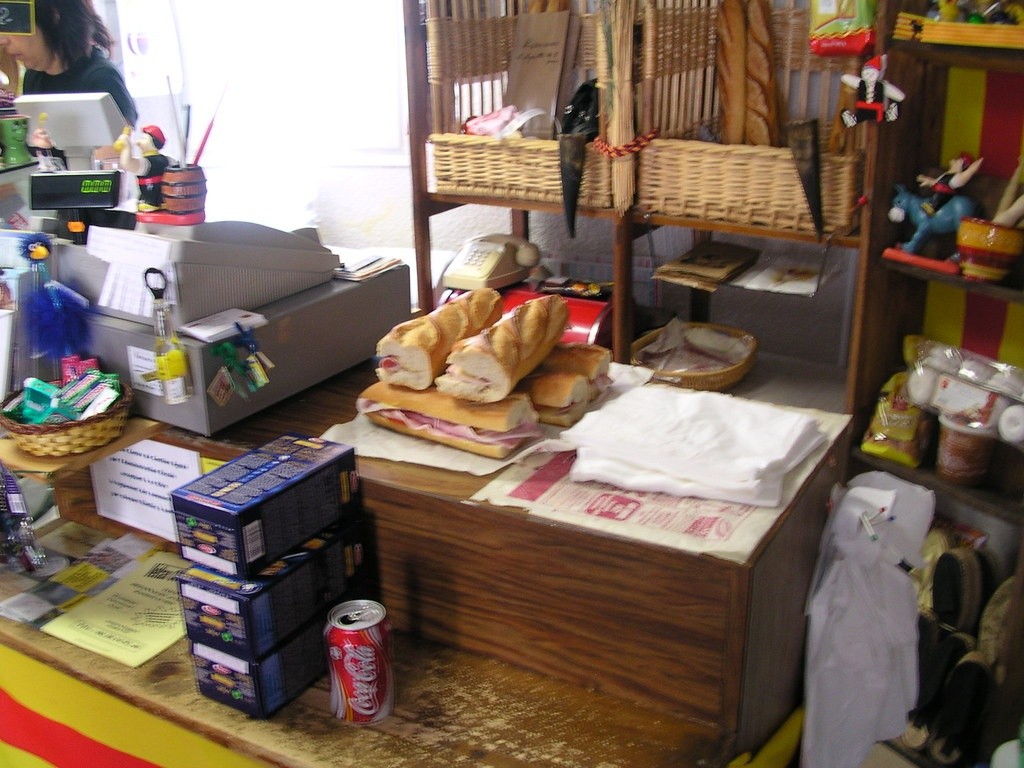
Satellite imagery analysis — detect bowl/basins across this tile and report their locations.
[958,216,1024,282]
[935,417,996,486]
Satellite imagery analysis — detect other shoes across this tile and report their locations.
[901,631,976,750]
[929,649,993,765]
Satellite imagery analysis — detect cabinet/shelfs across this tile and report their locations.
[850,0,1024,581]
[403,0,951,442]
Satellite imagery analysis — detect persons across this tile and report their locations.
[118,125,165,211]
[0,0,138,129]
[917,152,984,214]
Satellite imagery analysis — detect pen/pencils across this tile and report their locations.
[164,74,229,170]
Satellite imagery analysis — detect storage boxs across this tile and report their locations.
[175,504,380,658]
[170,430,362,580]
[189,569,385,722]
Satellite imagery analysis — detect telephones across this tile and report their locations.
[440,234,542,290]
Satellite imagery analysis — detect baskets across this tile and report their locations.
[0,372,136,459]
[636,0,864,236]
[630,321,758,392]
[426,0,617,208]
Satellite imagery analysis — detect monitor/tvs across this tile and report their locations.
[13,92,134,171]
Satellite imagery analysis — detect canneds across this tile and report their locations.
[323,600,396,726]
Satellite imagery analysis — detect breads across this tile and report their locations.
[525,0,569,14]
[714,0,781,149]
[354,287,612,459]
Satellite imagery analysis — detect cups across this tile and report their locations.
[0,116,32,163]
[162,164,207,214]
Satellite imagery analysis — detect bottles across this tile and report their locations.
[145,268,192,406]
